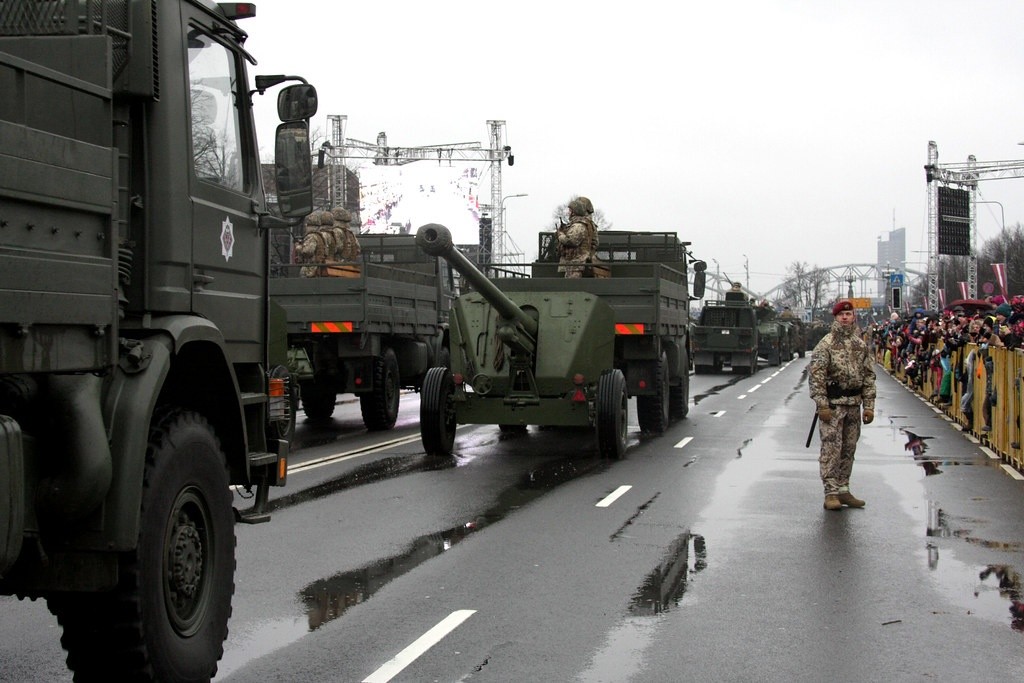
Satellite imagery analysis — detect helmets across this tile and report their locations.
[333,206,352,222]
[568,201,587,216]
[321,211,334,225]
[305,213,321,227]
[575,197,594,213]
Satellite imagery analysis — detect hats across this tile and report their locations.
[995,303,1012,317]
[832,301,854,314]
[954,305,964,311]
[989,296,1004,306]
[983,317,993,327]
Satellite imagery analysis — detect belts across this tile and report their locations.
[829,387,863,397]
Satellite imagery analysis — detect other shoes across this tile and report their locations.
[841,491,866,508]
[982,425,993,432]
[962,425,974,432]
[929,393,952,406]
[823,494,842,511]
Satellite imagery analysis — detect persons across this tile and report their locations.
[870,295,1024,449]
[294,207,361,278]
[781,306,795,318]
[556,196,599,278]
[809,301,877,509]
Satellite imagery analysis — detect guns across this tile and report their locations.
[290,232,304,242]
[559,216,566,228]
[805,404,819,448]
[722,271,734,287]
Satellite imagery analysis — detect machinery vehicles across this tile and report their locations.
[687,294,805,376]
[525,228,708,437]
[267,225,462,431]
[0,0,319,683]
[411,221,636,463]
